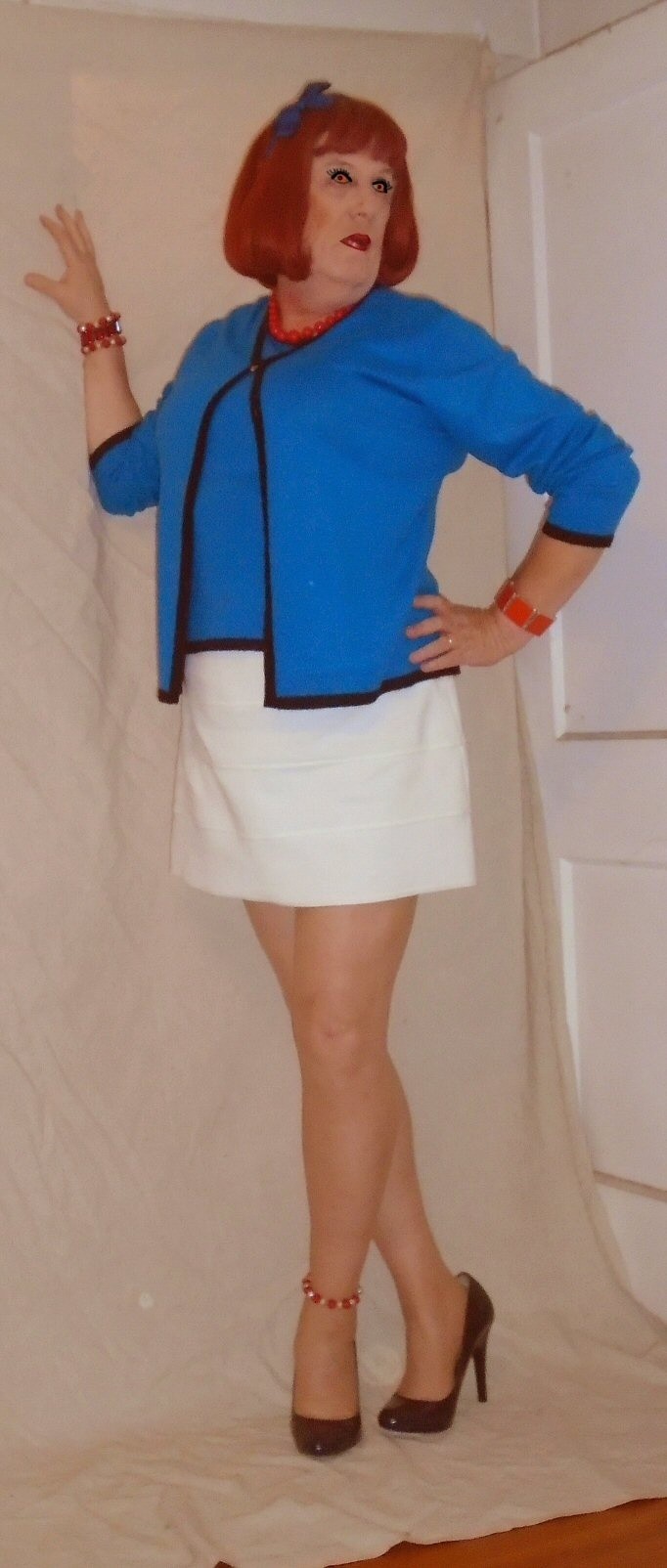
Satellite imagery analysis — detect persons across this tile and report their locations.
[24,83,640,1457]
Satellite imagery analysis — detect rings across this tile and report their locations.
[441,633,454,648]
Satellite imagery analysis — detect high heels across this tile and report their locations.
[380,1270,491,1431]
[290,1340,363,1457]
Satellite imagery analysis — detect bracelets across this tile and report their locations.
[79,313,127,354]
[494,579,556,638]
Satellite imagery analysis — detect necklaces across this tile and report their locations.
[268,286,354,343]
